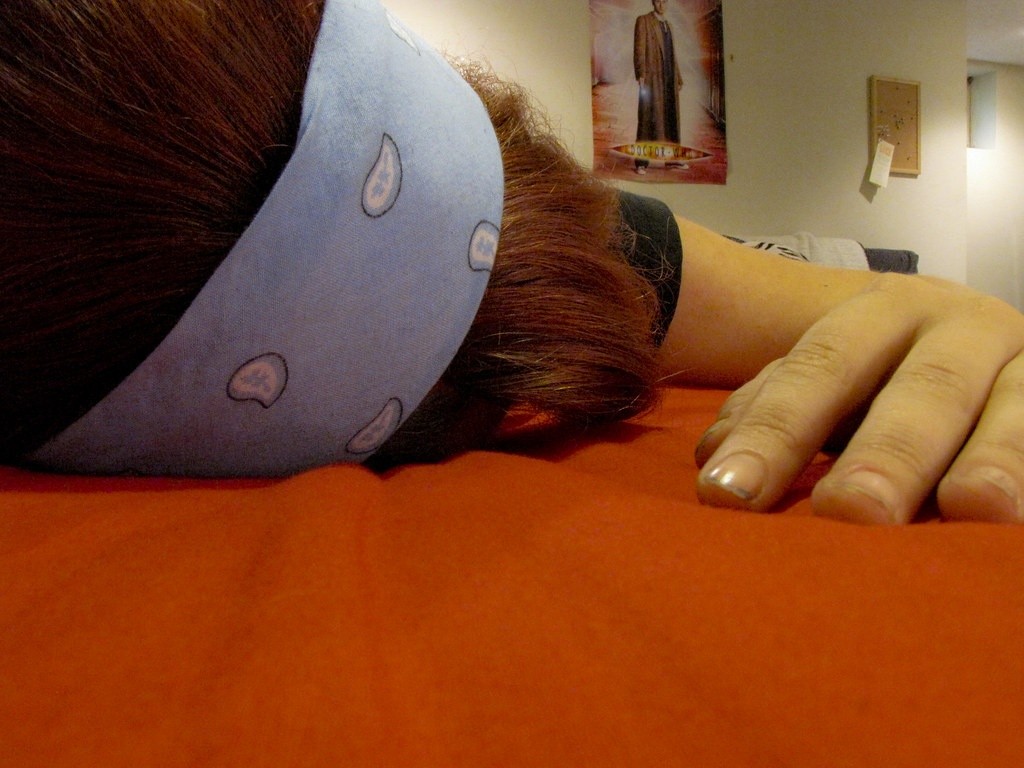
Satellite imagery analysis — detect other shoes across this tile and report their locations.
[637,166,646,175]
[665,163,689,169]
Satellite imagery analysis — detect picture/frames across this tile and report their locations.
[870,75,921,175]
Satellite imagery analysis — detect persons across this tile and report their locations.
[635,0,690,174]
[1,0,1024,525]
